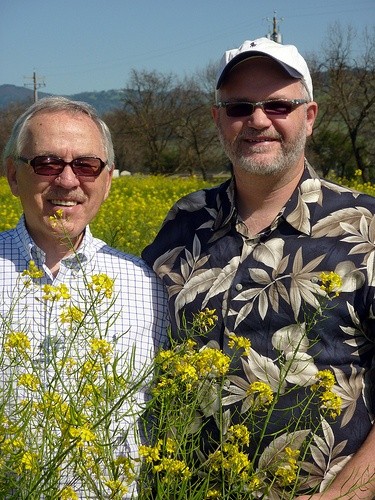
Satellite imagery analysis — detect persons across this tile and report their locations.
[141,37,375,500]
[0,96,171,500]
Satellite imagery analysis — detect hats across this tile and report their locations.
[216,37,313,102]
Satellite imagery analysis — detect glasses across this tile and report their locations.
[217,99,308,117]
[17,155,109,176]
